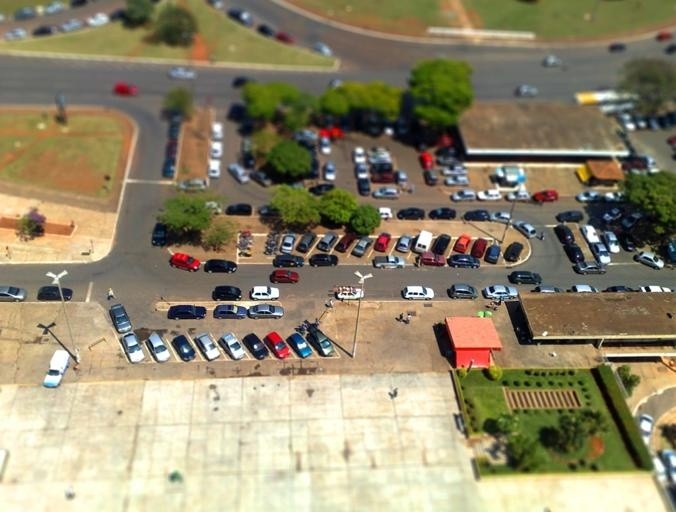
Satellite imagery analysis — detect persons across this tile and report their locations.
[399,313,404,322]
[540,232,544,241]
[329,298,334,309]
[298,317,320,332]
[407,313,411,324]
[107,287,116,301]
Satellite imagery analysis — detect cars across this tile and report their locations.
[266,331,290,359]
[170,335,196,362]
[449,254,481,269]
[1,284,74,303]
[167,304,207,321]
[606,285,633,292]
[308,253,338,267]
[274,254,305,268]
[574,261,607,276]
[250,285,280,301]
[419,253,446,267]
[170,253,201,272]
[638,285,672,292]
[637,252,665,271]
[121,330,145,363]
[535,285,564,292]
[109,303,133,333]
[449,283,478,300]
[213,305,247,319]
[195,332,221,361]
[336,286,365,301]
[151,110,676,265]
[287,333,313,359]
[204,259,237,274]
[403,285,435,300]
[214,285,242,301]
[571,284,599,292]
[374,255,406,269]
[309,329,334,357]
[271,269,299,284]
[145,333,170,363]
[242,333,269,360]
[484,284,517,300]
[517,31,675,97]
[509,271,542,285]
[2,0,333,96]
[638,413,676,492]
[220,332,246,360]
[248,303,284,320]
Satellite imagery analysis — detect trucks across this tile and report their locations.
[43,349,69,388]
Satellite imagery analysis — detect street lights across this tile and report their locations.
[352,270,373,357]
[45,269,80,364]
